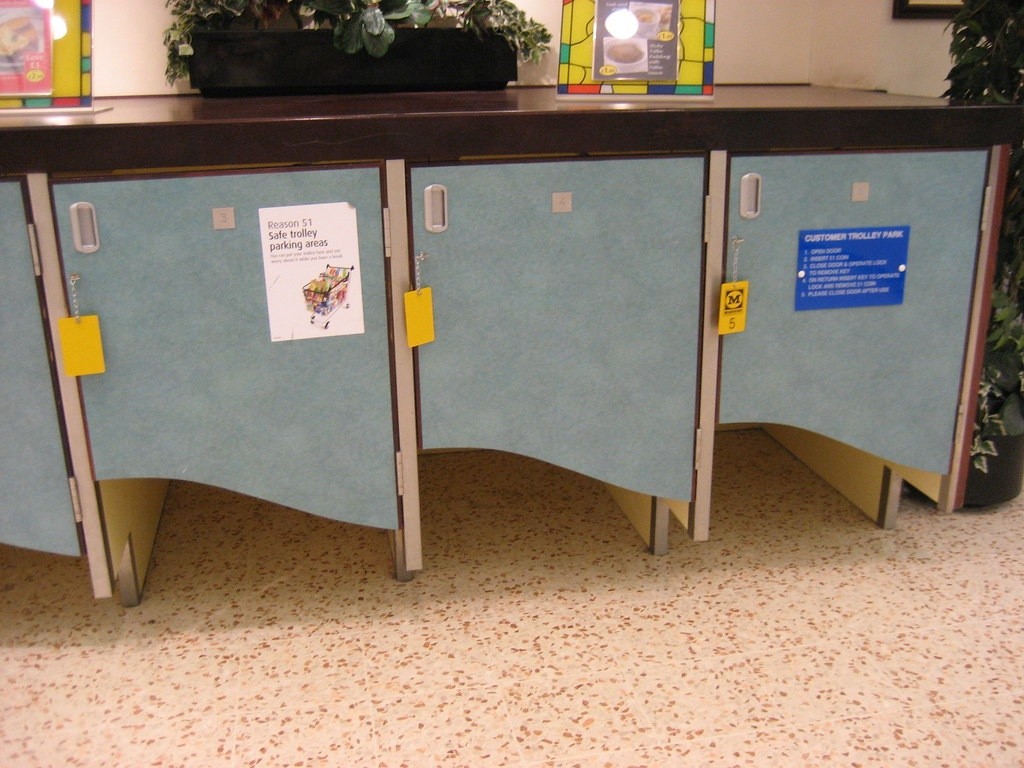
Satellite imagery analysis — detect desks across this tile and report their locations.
[1,82,1023,608]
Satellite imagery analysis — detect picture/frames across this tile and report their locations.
[893,0,966,20]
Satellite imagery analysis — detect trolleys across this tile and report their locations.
[301,263,355,328]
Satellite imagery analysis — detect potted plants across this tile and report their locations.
[939,0,1024,508]
[163,1,554,98]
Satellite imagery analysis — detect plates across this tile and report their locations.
[602,39,647,67]
[0,16,43,67]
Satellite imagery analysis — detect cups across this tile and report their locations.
[633,7,660,34]
